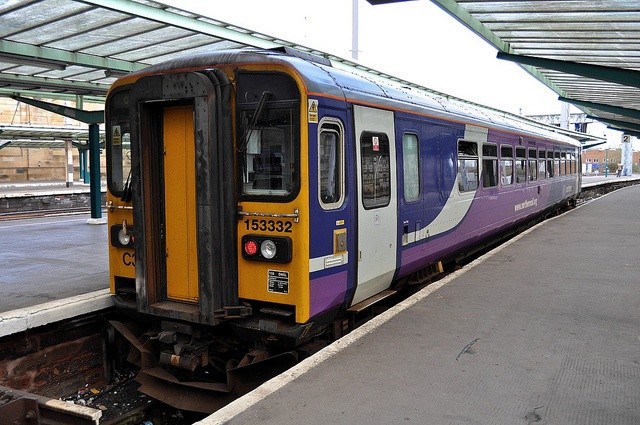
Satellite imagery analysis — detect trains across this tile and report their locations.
[106,48,584,415]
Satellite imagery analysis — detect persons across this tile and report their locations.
[616,162,623,177]
